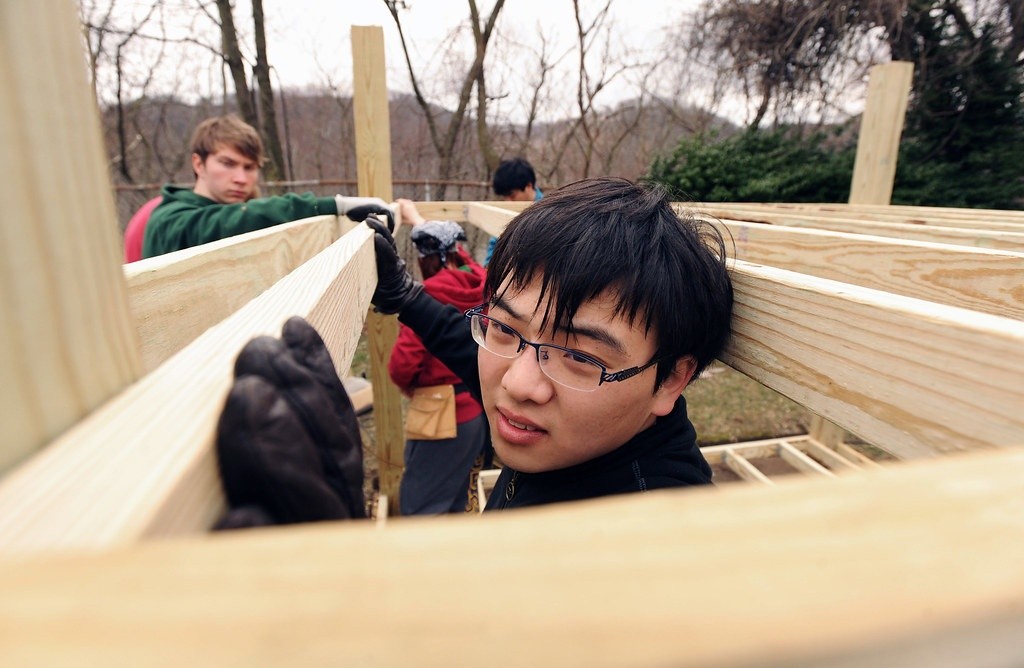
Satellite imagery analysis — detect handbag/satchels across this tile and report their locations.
[405,383,468,440]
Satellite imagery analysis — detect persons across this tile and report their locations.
[139,113,397,262]
[483,157,546,268]
[213,175,734,534]
[384,196,496,515]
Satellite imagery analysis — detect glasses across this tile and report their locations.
[465,301,663,392]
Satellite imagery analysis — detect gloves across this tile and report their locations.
[209,316,366,532]
[366,214,425,315]
[336,194,395,234]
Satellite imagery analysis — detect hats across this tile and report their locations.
[412,220,468,261]
